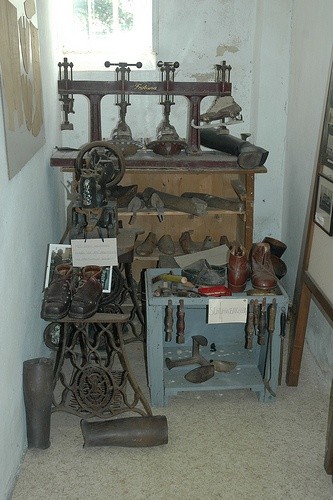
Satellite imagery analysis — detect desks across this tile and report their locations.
[44,298,153,422]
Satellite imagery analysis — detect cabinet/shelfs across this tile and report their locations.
[50,81,267,307]
[144,268,290,408]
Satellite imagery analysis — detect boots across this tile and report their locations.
[198,125,270,168]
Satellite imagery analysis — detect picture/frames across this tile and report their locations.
[313,173,333,236]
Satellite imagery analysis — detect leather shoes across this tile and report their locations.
[69,265,102,318]
[41,263,75,319]
[227,242,248,290]
[250,243,277,291]
